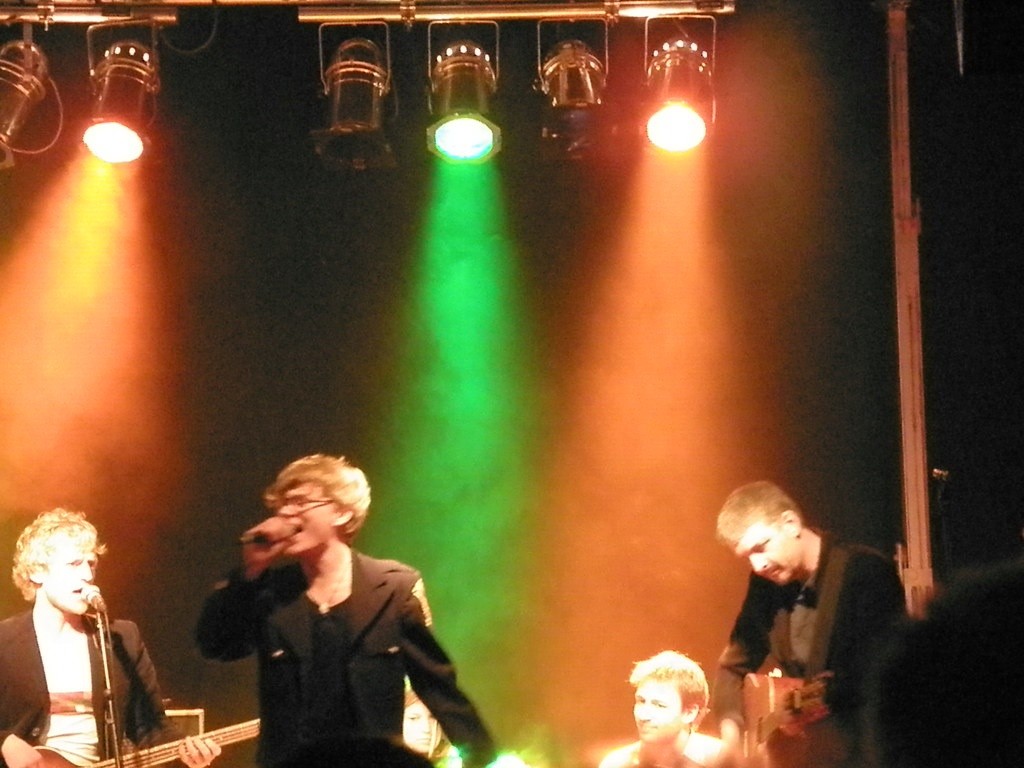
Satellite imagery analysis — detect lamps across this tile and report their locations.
[82,38,160,165]
[0,39,51,169]
[425,40,502,166]
[307,38,399,173]
[531,38,618,160]
[639,36,713,152]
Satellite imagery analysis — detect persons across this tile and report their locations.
[0,506,222,768]
[195,453,496,768]
[717,481,917,768]
[600,650,723,768]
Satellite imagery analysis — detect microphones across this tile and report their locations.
[80,585,106,612]
[237,534,266,545]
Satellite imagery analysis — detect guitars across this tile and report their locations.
[740,672,829,759]
[28,717,260,768]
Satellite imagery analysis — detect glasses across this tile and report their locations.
[264,493,337,510]
